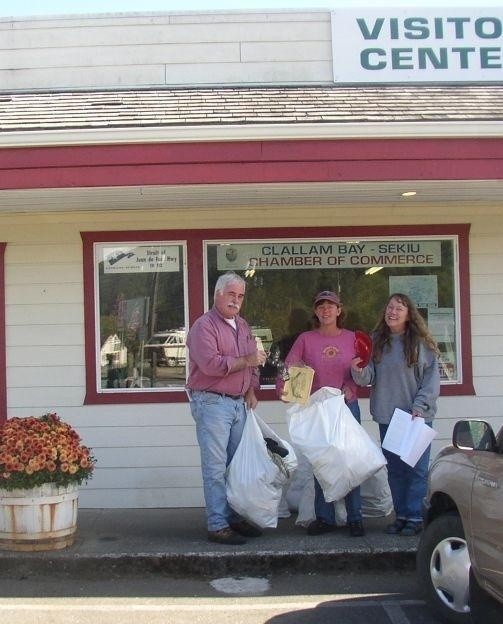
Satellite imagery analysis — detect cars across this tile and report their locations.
[413,417,502,623]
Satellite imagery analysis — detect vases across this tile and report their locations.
[0,484,79,552]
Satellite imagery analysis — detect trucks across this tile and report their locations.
[142,325,273,367]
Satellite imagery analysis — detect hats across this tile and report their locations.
[313,290,341,307]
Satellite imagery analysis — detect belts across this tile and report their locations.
[209,391,242,400]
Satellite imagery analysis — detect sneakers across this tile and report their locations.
[400,520,423,537]
[386,518,405,535]
[207,526,247,545]
[229,519,263,538]
[346,518,365,537]
[308,519,336,535]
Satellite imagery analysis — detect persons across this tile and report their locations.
[184,273,266,544]
[278,290,365,537]
[351,292,441,538]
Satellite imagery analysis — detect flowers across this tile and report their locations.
[0,410,97,487]
